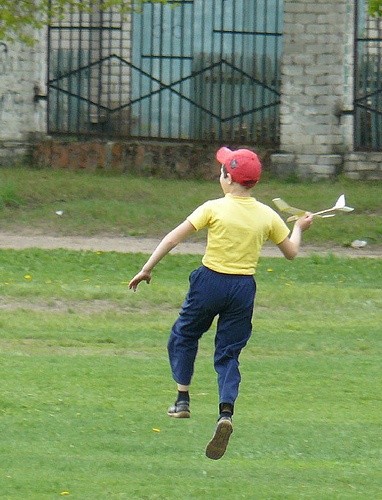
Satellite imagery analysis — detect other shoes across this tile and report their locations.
[205,417,234,460]
[167,400,190,418]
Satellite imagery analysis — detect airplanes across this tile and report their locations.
[272,194,355,223]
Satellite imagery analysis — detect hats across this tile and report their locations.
[216,147,261,188]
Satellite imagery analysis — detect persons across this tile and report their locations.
[127,146,315,460]
